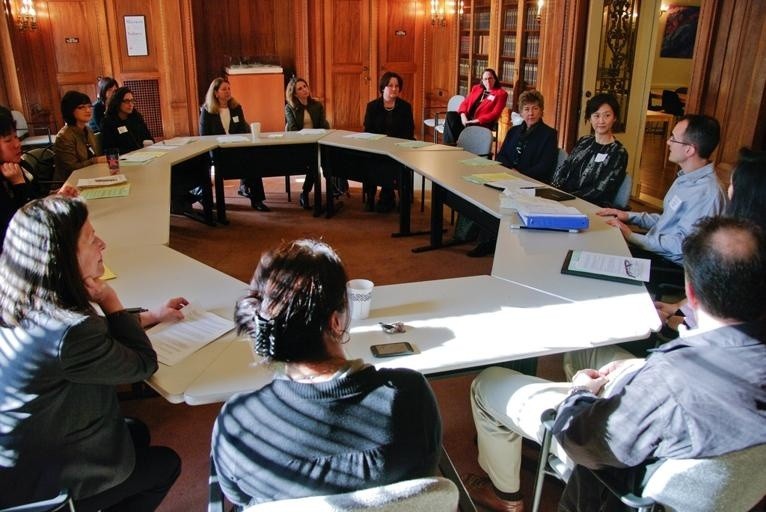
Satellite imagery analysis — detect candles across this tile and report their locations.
[534,0,544,24]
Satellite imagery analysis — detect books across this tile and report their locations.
[459,7,541,109]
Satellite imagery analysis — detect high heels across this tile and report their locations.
[299,193,310,210]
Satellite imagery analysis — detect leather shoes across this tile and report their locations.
[449,237,478,246]
[461,473,525,512]
[250,201,272,212]
[237,184,251,198]
[172,193,205,212]
[184,208,200,215]
[465,240,496,258]
[331,184,342,198]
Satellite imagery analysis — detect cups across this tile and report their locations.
[143,140,152,148]
[104,148,120,176]
[251,123,261,141]
[347,278,374,319]
[225,55,273,70]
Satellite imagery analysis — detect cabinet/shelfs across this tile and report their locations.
[449,0,499,104]
[492,0,566,132]
[224,66,286,134]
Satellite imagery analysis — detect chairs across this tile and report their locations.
[641,86,688,173]
[206,446,479,512]
[525,395,765,511]
[10,107,60,172]
[421,95,466,143]
[0,487,78,510]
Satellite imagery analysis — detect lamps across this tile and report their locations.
[13,0,41,30]
[427,0,447,35]
[658,4,670,18]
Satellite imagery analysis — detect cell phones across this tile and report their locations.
[369,341,415,358]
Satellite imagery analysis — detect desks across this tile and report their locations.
[56,128,663,407]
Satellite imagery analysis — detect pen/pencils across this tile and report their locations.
[95,180,117,182]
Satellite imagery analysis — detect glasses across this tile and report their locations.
[625,259,636,278]
[77,104,95,111]
[122,98,137,105]
[669,138,689,145]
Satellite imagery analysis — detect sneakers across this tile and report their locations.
[376,195,394,213]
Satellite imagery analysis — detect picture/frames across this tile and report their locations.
[121,15,150,58]
